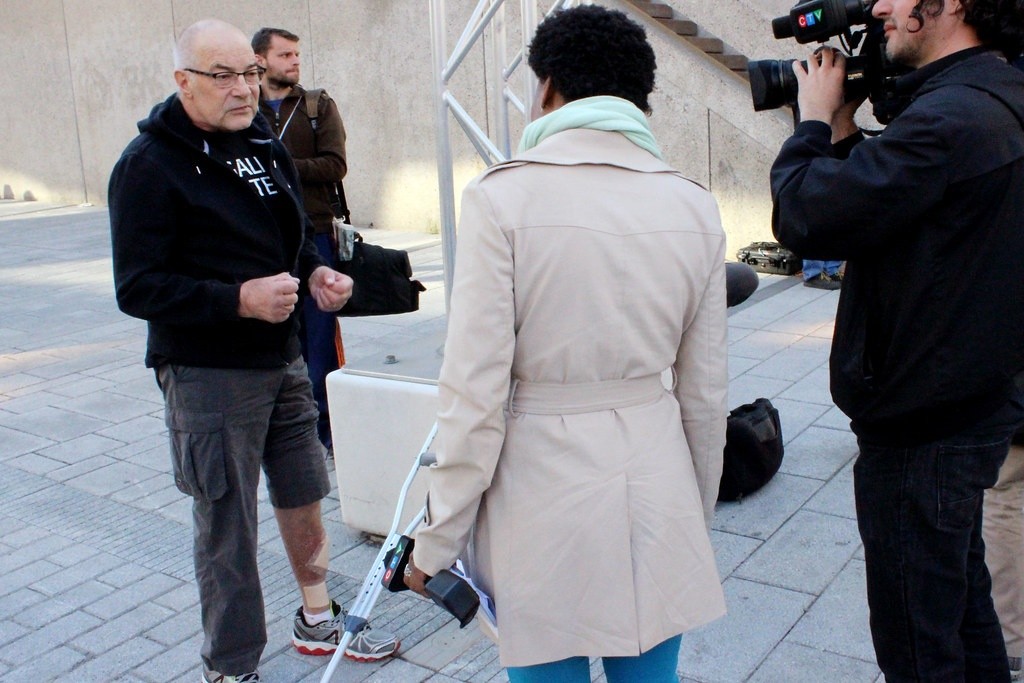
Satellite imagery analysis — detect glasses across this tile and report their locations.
[183,65,266,88]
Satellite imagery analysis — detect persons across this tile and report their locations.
[108,18,403,683]
[404,4,729,683]
[251,26,348,460]
[769,0,1024,683]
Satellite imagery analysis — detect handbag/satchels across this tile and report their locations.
[330,218,427,317]
[716,398,783,502]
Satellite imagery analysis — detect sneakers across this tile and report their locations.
[803,272,844,290]
[201,661,266,683]
[292,599,401,661]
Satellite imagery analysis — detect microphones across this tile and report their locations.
[381,535,481,629]
[771,15,794,40]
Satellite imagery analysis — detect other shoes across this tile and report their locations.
[1008,656,1021,678]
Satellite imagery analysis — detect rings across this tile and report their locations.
[404,564,411,578]
